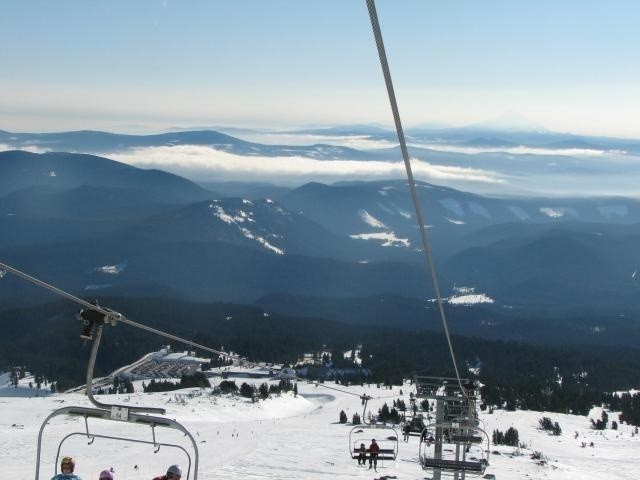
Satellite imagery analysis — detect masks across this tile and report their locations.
[63,470,71,474]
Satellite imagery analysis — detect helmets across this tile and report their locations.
[167,464,182,476]
[100,470,113,479]
[61,457,74,464]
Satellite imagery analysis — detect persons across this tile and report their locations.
[421,425,428,444]
[50,454,83,480]
[403,421,410,443]
[150,464,183,480]
[357,442,366,466]
[97,469,115,480]
[368,439,380,473]
